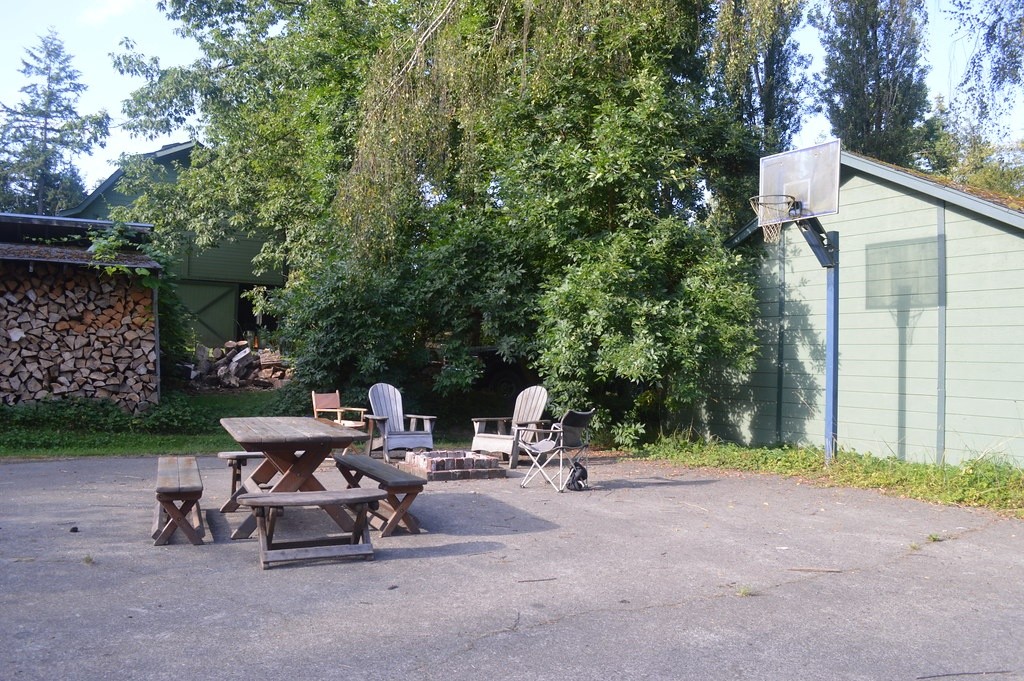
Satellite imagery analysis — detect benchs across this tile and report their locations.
[151,456,205,547]
[217,450,305,504]
[237,488,388,570]
[333,454,427,536]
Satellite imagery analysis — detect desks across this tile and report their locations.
[220,417,370,540]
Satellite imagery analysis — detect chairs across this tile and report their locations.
[510,408,596,493]
[364,382,437,463]
[311,389,368,460]
[471,386,549,468]
